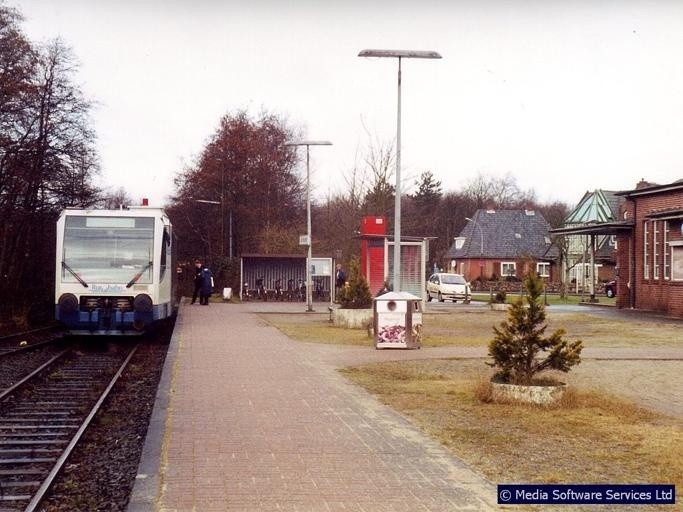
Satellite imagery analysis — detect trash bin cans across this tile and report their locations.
[223,287,233,300]
[374,291,424,350]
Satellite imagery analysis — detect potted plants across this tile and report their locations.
[484,269,585,405]
[337,257,374,329]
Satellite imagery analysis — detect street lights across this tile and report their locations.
[284,140,333,312]
[465,218,483,290]
[357,49,443,349]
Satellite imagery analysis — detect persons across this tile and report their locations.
[333,263,346,303]
[190,259,205,304]
[193,265,212,305]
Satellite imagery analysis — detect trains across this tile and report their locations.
[54,198,178,337]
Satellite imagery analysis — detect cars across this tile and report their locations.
[604,280,617,298]
[426,273,471,304]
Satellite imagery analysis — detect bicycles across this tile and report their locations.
[242,275,325,302]
